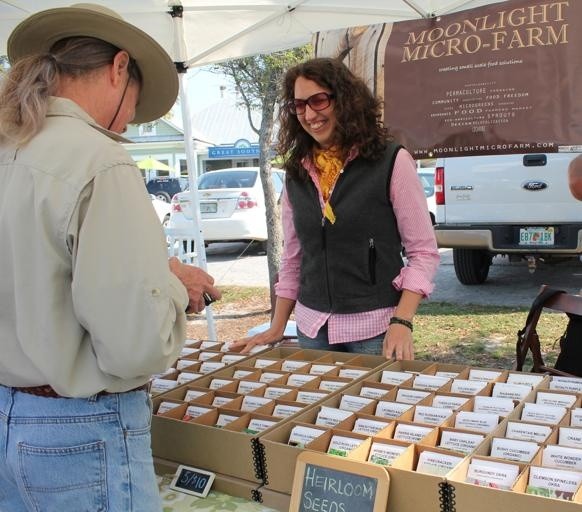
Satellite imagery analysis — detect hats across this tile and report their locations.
[152,195,172,245]
[417,167,436,225]
[170,167,285,254]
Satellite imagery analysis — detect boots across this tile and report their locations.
[145,175,187,202]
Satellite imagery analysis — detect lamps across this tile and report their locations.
[510,284,581,378]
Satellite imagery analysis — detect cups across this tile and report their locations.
[287,90,333,116]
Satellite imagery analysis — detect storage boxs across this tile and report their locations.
[147,339,582,512]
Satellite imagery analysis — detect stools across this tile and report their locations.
[13,377,153,400]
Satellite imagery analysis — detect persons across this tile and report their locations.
[227,58,440,361]
[0,3,223,512]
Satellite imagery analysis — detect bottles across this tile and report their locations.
[4,2,180,129]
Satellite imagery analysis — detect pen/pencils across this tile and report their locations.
[433,152,581,284]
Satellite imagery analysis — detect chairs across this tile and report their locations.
[510,284,581,378]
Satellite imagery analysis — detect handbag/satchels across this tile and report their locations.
[389,317,414,333]
